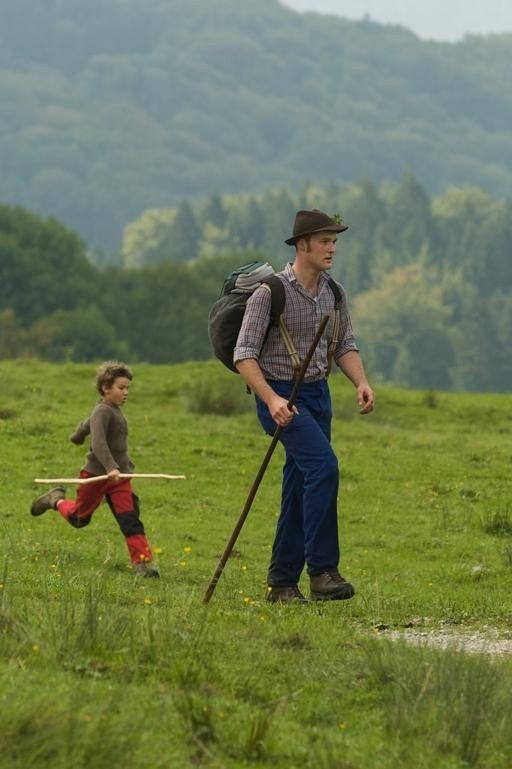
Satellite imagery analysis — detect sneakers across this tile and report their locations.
[137,569,160,578]
[30,486,65,516]
[309,571,355,601]
[265,585,313,605]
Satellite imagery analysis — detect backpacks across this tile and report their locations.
[207,260,342,374]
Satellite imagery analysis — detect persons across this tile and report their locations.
[30,362,160,578]
[233,208,375,604]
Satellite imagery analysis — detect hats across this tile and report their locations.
[284,209,349,246]
